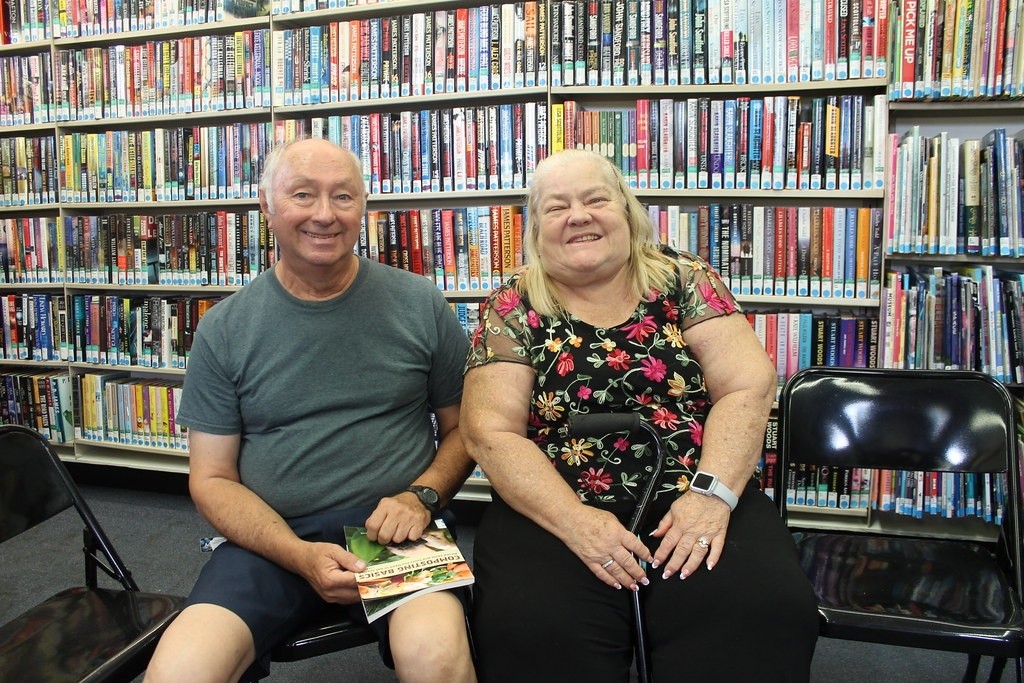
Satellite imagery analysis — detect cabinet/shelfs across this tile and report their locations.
[0,0,1024,546]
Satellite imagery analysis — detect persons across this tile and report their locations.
[141,136,480,683]
[456,148,824,683]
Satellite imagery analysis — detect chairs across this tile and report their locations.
[0,425,191,683]
[774,365,1024,682]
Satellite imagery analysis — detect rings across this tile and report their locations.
[696,537,711,549]
[602,559,613,570]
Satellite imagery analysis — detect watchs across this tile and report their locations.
[402,485,442,519]
[690,470,738,511]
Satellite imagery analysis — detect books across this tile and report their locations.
[343,520,475,623]
[0,0,1024,524]
